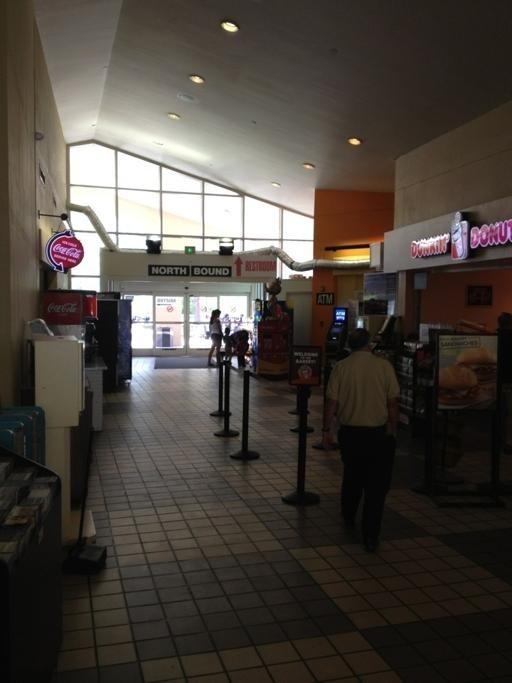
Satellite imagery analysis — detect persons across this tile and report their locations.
[204,311,250,368]
[320,328,401,552]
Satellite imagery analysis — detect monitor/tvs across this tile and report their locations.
[332,307,348,323]
[362,271,429,318]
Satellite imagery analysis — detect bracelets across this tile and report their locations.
[322,428,330,432]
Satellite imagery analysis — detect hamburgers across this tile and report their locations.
[438,346,497,405]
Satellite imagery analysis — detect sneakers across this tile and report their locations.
[208,362,220,367]
